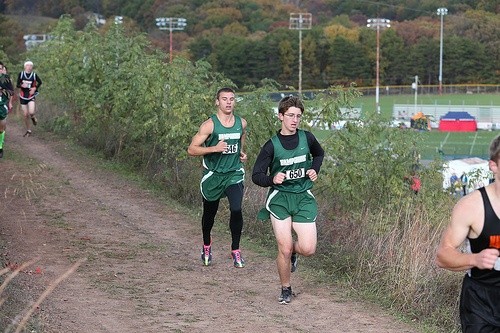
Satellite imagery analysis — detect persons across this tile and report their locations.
[405,168,468,197]
[0,59,42,158]
[436,133,500,333]
[187,87,247,268]
[252,96,325,304]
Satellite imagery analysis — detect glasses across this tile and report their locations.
[284,113,302,119]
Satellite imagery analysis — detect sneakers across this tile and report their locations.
[0,149,3,158]
[290,252,297,272]
[230,246,245,268]
[23,131,32,137]
[278,286,291,305]
[201,238,212,266]
[31,117,37,126]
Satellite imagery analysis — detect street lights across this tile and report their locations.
[156,17,187,63]
[367,18,390,113]
[437,7,448,94]
